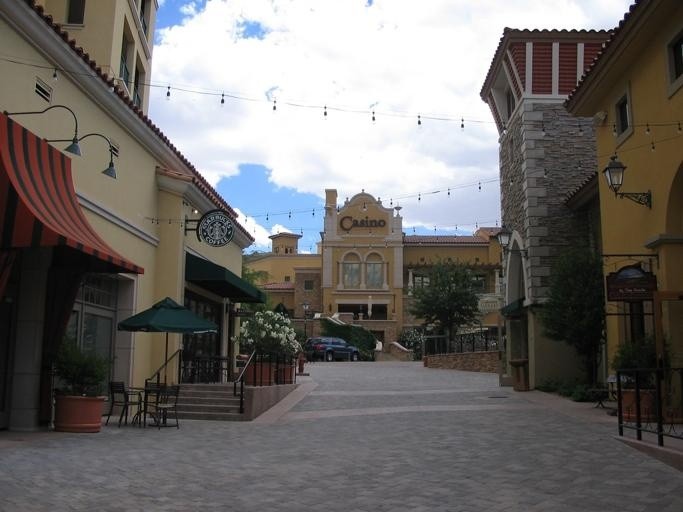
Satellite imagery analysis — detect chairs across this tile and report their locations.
[105,380,183,430]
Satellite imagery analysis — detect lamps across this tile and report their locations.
[602,153,652,210]
[5,104,117,179]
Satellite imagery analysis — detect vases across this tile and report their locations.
[245,364,294,385]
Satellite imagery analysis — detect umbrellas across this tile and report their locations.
[118,297,219,424]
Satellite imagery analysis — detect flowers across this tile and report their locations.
[230,311,302,363]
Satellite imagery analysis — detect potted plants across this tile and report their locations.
[52,353,114,435]
[611,335,670,423]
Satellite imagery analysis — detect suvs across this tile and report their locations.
[304,335,360,363]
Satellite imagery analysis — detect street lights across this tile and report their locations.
[300,300,311,342]
